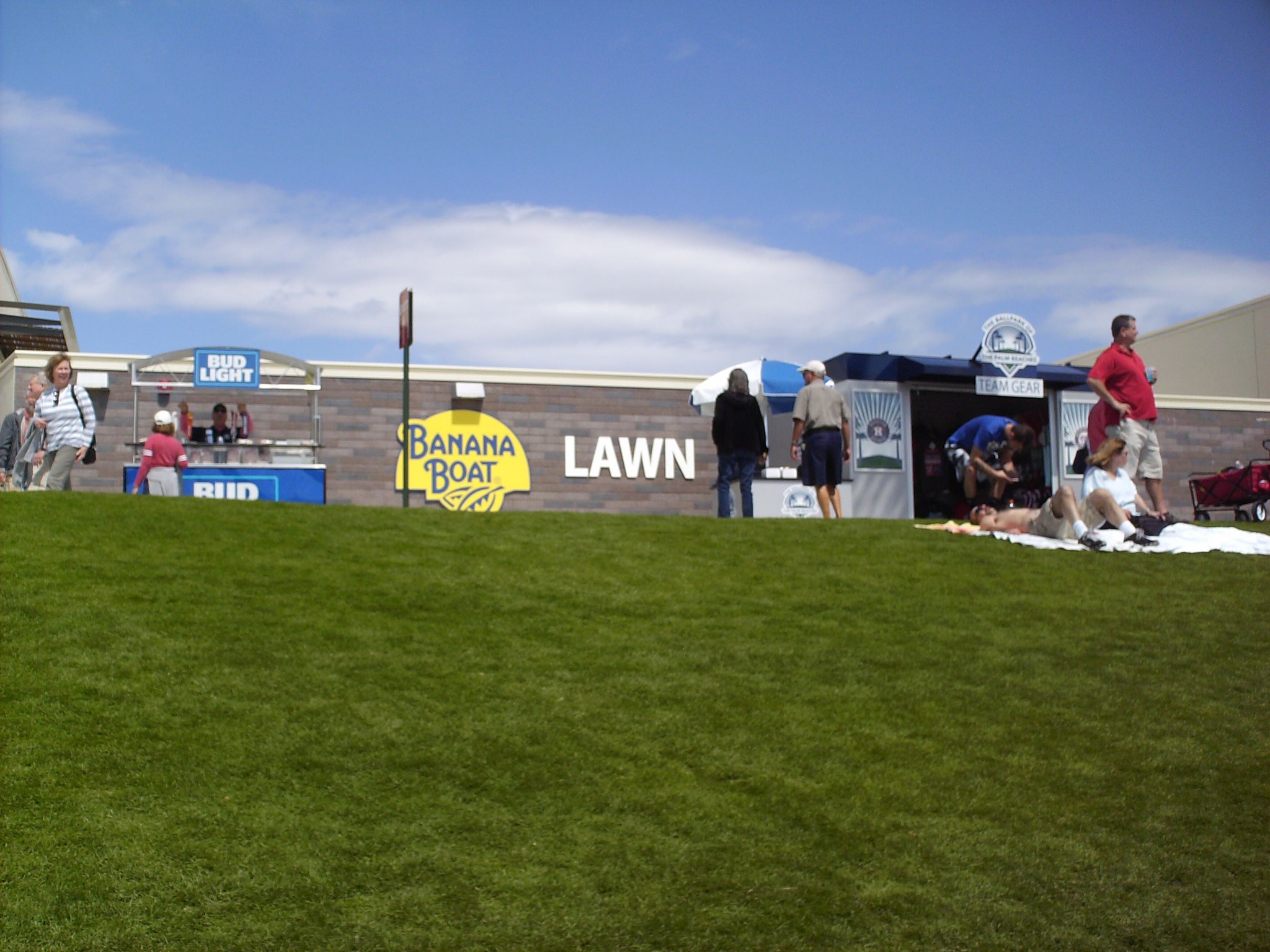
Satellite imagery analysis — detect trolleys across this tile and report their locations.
[1188,439,1270,522]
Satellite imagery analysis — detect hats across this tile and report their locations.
[212,402,227,413]
[796,359,825,373]
[153,410,174,425]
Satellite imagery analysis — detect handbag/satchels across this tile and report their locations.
[80,433,97,464]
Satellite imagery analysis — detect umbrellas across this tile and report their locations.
[688,358,834,468]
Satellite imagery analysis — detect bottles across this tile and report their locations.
[1006,499,1016,510]
[1235,460,1244,469]
[239,412,248,438]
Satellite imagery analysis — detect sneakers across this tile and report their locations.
[1124,532,1161,546]
[1078,532,1106,549]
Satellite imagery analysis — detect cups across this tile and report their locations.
[1144,367,1156,382]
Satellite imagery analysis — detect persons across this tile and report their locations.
[1086,315,1188,523]
[790,360,851,519]
[33,354,95,491]
[178,400,253,442]
[968,485,1159,549]
[945,415,1034,512]
[27,371,55,491]
[0,390,37,489]
[1081,438,1167,536]
[131,410,188,497]
[712,369,766,517]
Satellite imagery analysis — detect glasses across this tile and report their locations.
[975,505,983,514]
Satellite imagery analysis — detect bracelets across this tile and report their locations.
[792,442,797,446]
[1144,509,1149,515]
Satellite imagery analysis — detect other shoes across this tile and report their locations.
[1160,512,1188,525]
